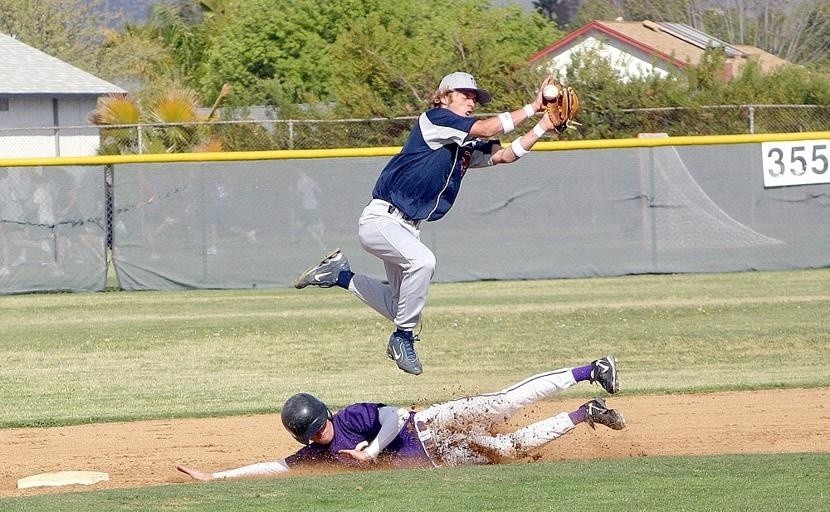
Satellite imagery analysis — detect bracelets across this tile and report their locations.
[532,122,546,139]
[522,102,535,120]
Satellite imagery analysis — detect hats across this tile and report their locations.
[439,71,490,103]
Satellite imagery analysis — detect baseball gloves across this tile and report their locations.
[544,77,577,132]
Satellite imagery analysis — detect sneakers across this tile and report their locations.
[386,332,423,375]
[295,248,349,289]
[580,399,625,430]
[590,356,619,394]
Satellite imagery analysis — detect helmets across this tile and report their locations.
[281,394,333,445]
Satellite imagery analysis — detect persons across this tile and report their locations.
[292,68,581,378]
[171,353,629,482]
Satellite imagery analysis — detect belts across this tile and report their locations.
[388,205,418,225]
[416,421,443,466]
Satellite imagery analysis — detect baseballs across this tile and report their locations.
[543,84,557,100]
